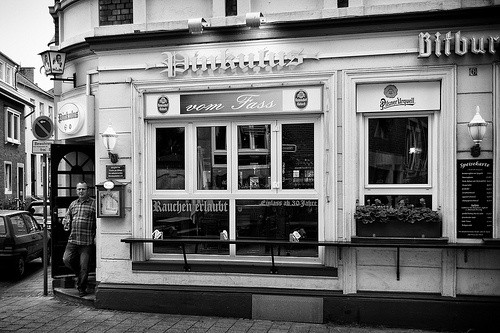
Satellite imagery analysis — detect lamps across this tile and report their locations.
[39,46,77,88]
[466,106,487,158]
[101,127,118,165]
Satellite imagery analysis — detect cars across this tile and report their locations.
[0,210,51,279]
[27,200,51,237]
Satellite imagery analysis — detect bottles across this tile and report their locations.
[64,211,69,230]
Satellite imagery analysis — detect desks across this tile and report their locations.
[156,216,193,243]
[122,237,500,282]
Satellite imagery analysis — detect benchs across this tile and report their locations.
[174,227,203,242]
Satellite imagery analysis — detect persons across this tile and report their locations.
[63,181,95,297]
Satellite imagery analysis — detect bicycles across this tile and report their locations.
[6,198,28,211]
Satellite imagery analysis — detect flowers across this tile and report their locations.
[352,199,443,225]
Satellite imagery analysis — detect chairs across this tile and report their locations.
[12,224,18,234]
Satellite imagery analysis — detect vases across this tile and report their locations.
[357,218,443,238]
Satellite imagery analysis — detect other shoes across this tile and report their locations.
[78,289,87,297]
